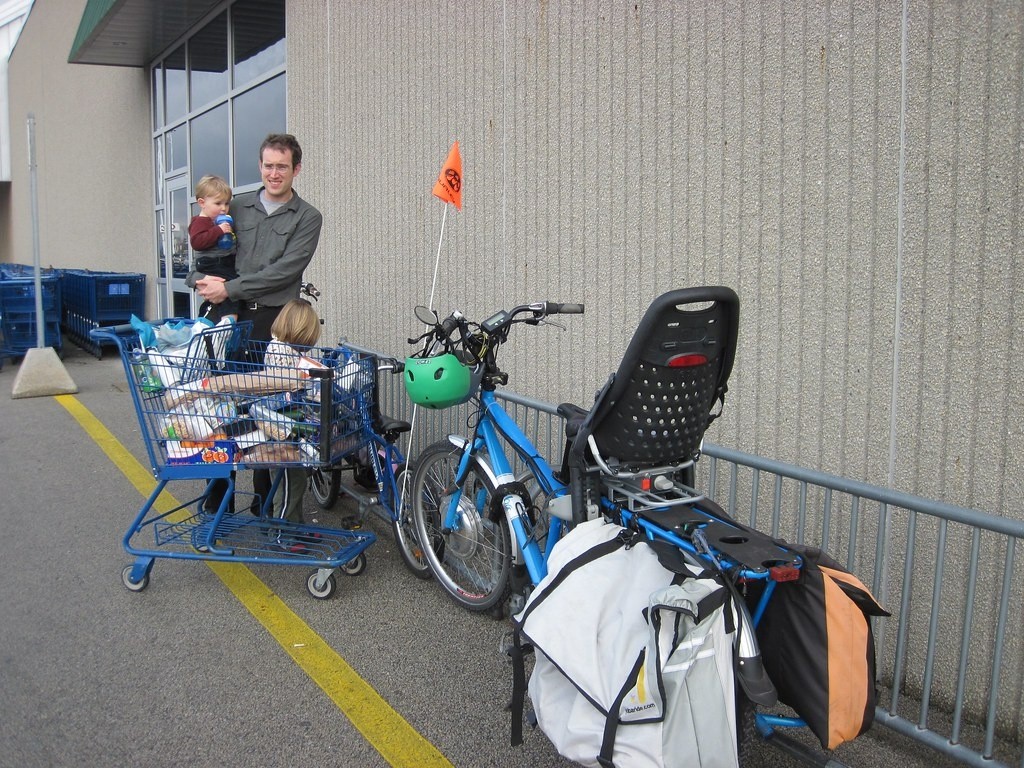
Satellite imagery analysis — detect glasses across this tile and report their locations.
[261,160,295,174]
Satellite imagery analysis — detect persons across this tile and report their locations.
[184,134,322,556]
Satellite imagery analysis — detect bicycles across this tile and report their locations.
[304,344,448,580]
[405,286,807,705]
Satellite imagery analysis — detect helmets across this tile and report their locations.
[404,350,485,409]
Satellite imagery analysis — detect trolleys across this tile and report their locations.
[0,261,147,371]
[89,316,379,603]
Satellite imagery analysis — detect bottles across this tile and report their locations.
[290,432,320,460]
[130,349,162,393]
[214,213,235,250]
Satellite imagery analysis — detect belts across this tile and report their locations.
[247,302,265,310]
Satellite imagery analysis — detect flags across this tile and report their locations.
[431,139,462,214]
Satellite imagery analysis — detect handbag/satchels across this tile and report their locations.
[130,312,232,390]
[511,517,740,768]
[699,499,892,750]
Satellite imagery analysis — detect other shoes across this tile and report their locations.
[260,515,274,534]
[298,531,321,543]
[279,539,307,553]
